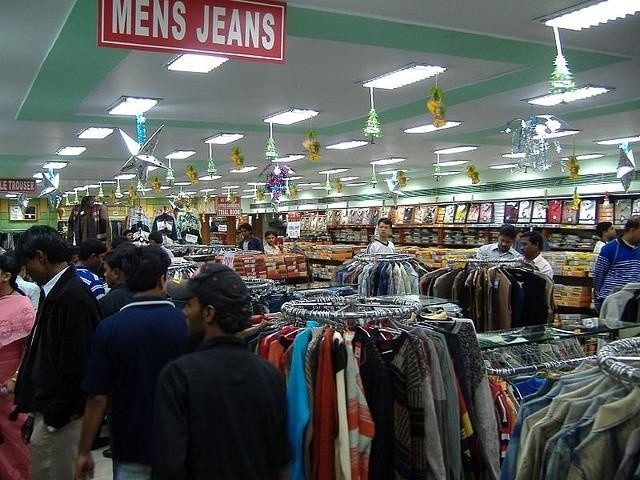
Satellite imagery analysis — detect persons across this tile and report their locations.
[476,223,555,281]
[365,218,394,254]
[593,221,617,255]
[593,215,640,323]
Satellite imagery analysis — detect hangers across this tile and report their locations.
[163,250,640,400]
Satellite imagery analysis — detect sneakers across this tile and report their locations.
[91,435,113,450]
[102,449,112,458]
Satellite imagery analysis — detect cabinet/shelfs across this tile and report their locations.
[198,210,237,248]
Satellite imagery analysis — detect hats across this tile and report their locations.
[165,264,253,322]
